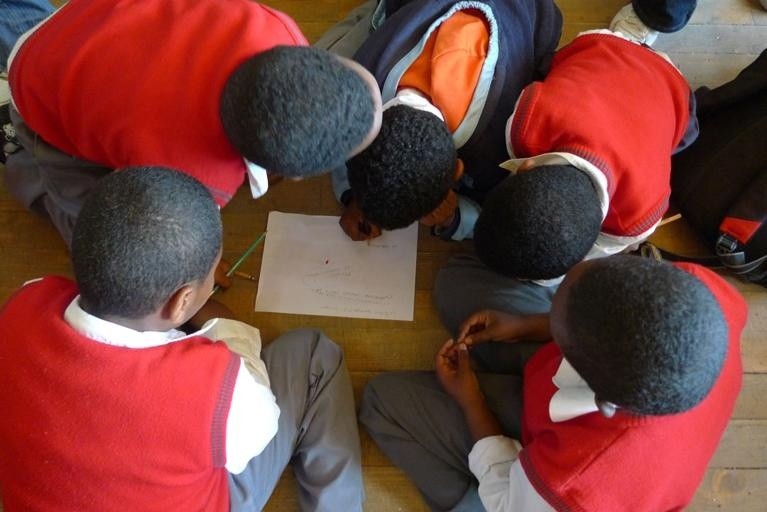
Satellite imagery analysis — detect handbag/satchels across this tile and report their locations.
[668,53,766,286]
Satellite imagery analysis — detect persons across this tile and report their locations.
[0,164,365,512]
[315,0,563,245]
[0,0,383,263]
[460,2,766,289]
[356,225,748,512]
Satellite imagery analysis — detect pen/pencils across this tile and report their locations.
[234,271,256,281]
[658,212,683,227]
[446,342,465,357]
[367,237,371,246]
[207,233,267,297]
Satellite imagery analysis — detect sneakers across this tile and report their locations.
[610,4,659,44]
